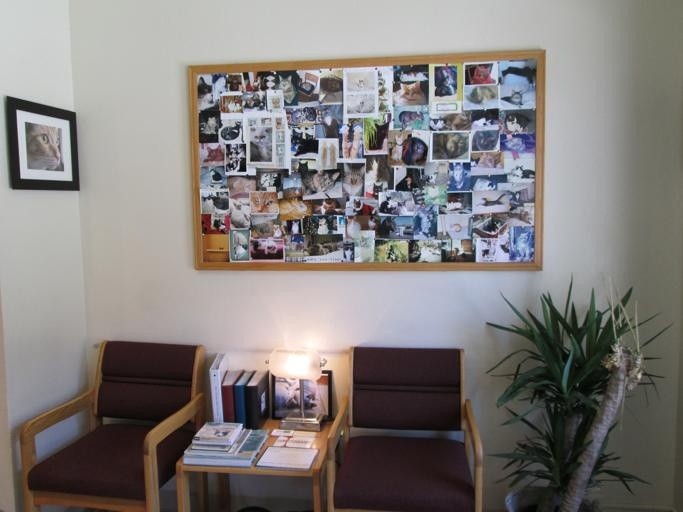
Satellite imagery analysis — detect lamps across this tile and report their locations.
[268,347,325,431]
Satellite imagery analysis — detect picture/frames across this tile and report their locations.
[270,368,333,421]
[189,47,545,271]
[6,95,80,192]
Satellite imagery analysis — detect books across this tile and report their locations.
[183,350,269,467]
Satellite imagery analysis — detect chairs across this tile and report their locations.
[325,345,484,512]
[20,340,209,512]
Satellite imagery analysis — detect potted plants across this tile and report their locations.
[486,271,674,512]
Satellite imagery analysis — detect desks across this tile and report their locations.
[176,417,339,512]
